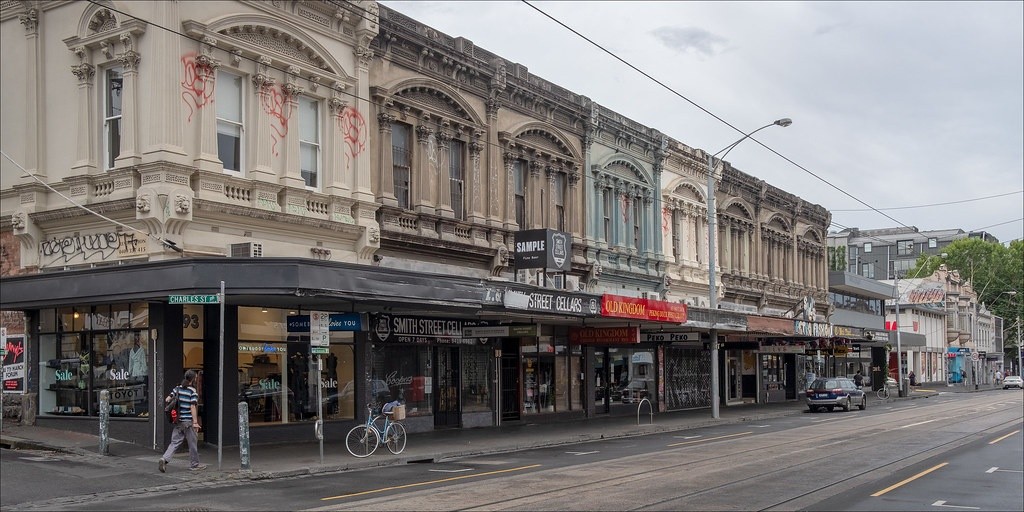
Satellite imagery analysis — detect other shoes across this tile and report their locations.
[190,464,206,471]
[158,458,166,473]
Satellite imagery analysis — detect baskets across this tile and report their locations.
[389,404,406,420]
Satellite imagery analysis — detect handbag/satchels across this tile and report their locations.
[164,386,180,425]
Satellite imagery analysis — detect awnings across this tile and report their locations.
[632,352,653,364]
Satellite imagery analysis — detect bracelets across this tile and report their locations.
[193,423,197,424]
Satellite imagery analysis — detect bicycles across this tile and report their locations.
[345,404,408,458]
[877,379,891,400]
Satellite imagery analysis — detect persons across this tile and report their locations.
[908,371,917,392]
[962,371,968,386]
[325,352,339,415]
[989,370,994,386]
[79,347,90,379]
[854,370,865,390]
[159,370,207,473]
[996,370,1001,385]
[127,343,148,377]
[1006,370,1010,376]
[307,353,319,417]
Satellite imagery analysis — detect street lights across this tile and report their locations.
[972,291,1018,389]
[707,118,793,419]
[894,252,950,397]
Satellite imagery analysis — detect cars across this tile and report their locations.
[805,376,867,412]
[238,381,295,416]
[622,378,655,403]
[322,379,393,414]
[1001,375,1024,390]
